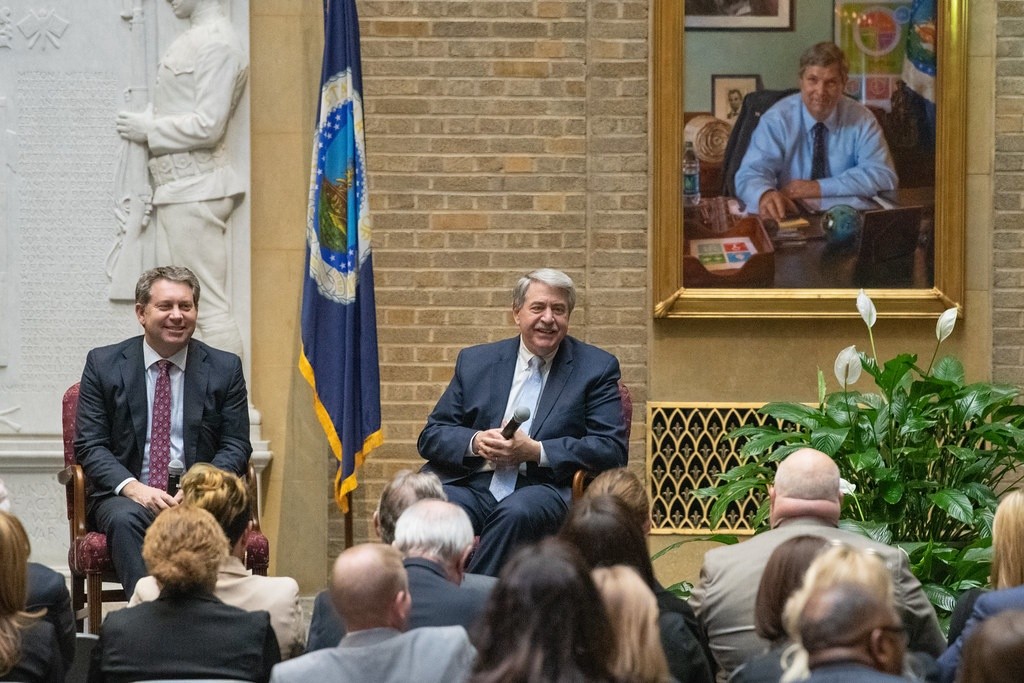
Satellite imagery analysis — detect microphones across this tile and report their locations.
[501,406,530,440]
[167,459,184,498]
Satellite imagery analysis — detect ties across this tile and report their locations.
[810,122,824,179]
[488,357,545,503]
[148,361,174,491]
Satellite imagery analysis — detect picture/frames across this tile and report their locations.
[711,73,760,124]
[654,0,970,322]
[685,0,793,31]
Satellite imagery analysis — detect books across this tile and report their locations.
[729,200,810,238]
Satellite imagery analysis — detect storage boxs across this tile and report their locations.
[683,217,774,286]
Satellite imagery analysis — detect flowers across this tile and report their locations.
[649,287,1023,638]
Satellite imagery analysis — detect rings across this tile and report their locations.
[496,456,498,461]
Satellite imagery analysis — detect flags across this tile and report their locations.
[297,0,385,513]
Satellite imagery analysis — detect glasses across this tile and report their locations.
[864,621,918,649]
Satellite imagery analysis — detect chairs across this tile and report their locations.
[59,382,268,635]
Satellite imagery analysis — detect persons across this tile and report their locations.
[415,267,630,578]
[0,477,77,683]
[87,502,281,683]
[726,535,922,683]
[734,41,899,223]
[373,467,498,589]
[578,466,651,537]
[125,463,306,662]
[303,499,489,652]
[73,266,253,603]
[557,493,722,682]
[117,0,249,366]
[924,488,1024,683]
[686,447,947,683]
[268,542,480,683]
[467,536,677,683]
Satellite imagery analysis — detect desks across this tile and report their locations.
[758,187,936,286]
